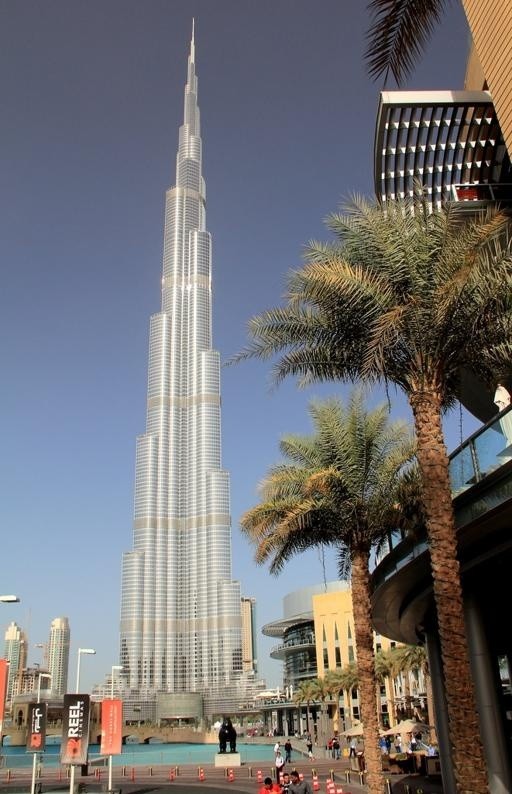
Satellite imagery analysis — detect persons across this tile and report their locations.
[285,739,293,763]
[302,729,308,741]
[259,777,281,793]
[325,733,422,760]
[280,772,292,794]
[294,729,301,738]
[288,770,313,794]
[354,740,366,773]
[273,741,280,756]
[306,740,315,761]
[274,750,284,783]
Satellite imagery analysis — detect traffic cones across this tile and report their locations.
[228,768,235,782]
[279,771,285,785]
[312,774,343,794]
[169,768,174,781]
[199,768,206,781]
[299,773,304,781]
[257,768,264,784]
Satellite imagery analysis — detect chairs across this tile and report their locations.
[353,751,441,776]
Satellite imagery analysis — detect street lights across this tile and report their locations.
[69,647,97,794]
[30,672,53,794]
[108,664,124,792]
